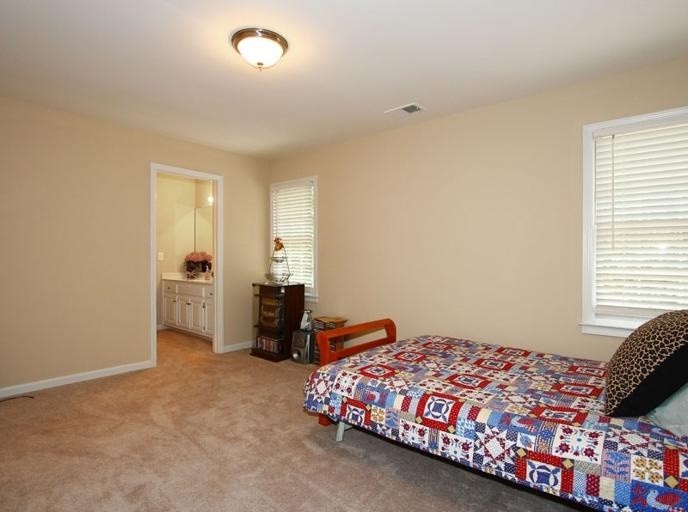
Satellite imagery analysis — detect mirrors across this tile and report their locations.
[194,206,212,274]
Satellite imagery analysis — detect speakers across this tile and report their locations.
[290,330,314,364]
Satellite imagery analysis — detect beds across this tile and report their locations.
[316,318,688,512]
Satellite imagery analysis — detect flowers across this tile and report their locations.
[184,251,212,273]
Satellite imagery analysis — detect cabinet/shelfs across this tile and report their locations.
[177,282,204,339]
[250,282,305,362]
[161,279,178,331]
[202,284,213,342]
[312,315,349,367]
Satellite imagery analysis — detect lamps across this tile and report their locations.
[230,27,290,71]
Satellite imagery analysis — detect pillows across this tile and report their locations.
[604,309,688,438]
